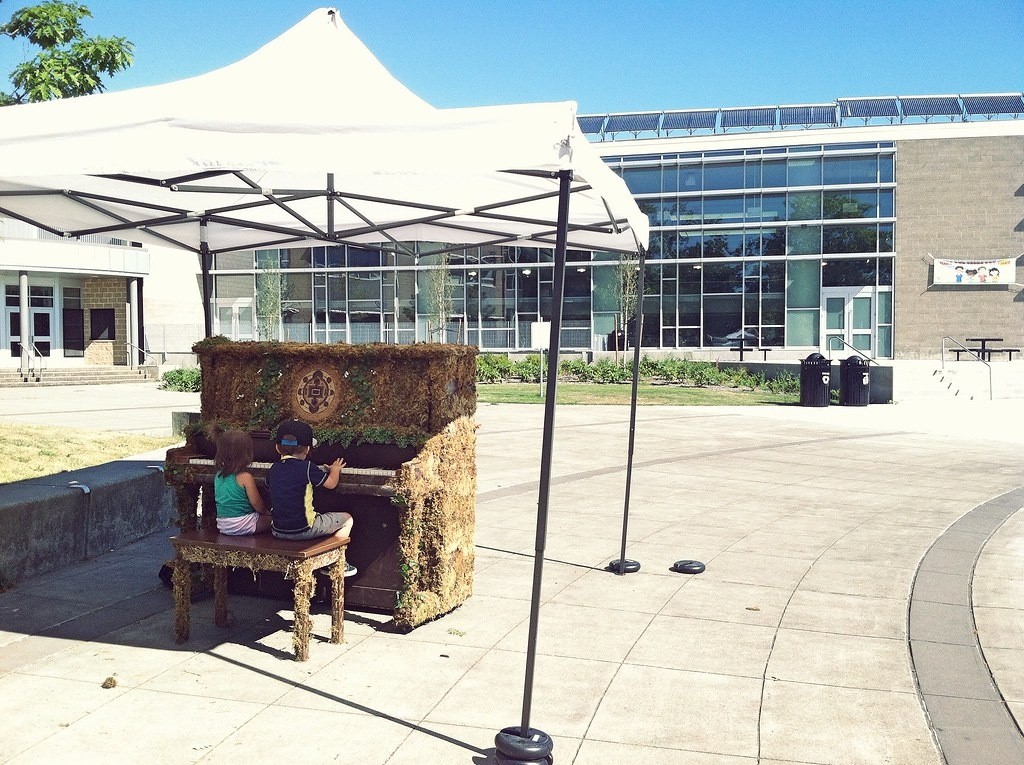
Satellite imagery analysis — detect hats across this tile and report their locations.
[274,419,317,448]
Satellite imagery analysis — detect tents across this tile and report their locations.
[0,6,651,752]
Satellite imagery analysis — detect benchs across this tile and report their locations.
[949,349,991,362]
[169,527,351,663]
[978,349,1020,361]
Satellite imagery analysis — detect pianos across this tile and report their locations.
[157,336,481,636]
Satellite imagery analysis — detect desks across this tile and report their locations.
[967,338,1003,361]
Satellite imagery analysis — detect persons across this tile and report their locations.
[264,420,358,577]
[207,421,272,536]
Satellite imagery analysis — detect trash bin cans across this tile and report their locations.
[798,353,834,407]
[837,356,871,406]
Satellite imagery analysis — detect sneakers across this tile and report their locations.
[321,557,357,577]
